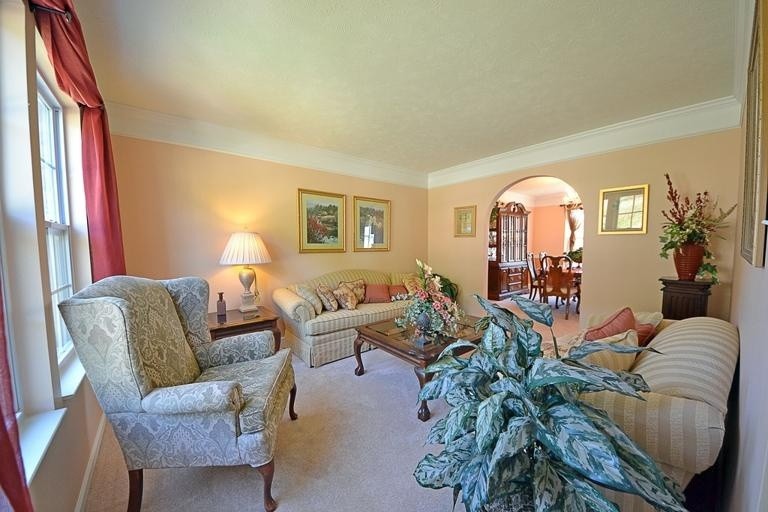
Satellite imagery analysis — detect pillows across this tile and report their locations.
[562,305,664,372]
[296,278,417,315]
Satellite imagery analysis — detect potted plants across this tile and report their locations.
[413,293,690,512]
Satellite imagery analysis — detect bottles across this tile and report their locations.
[217,315,226,327]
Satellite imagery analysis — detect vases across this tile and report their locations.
[739,0,768,268]
[416,310,432,346]
[673,240,704,281]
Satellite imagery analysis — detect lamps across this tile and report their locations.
[219,231,272,313]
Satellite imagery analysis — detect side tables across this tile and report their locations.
[207,306,281,352]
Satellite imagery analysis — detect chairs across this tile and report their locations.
[540,312,740,512]
[526,251,581,320]
[58,274,298,512]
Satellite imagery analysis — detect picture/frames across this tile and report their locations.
[352,195,391,252]
[453,205,476,237]
[297,187,347,253]
[597,184,649,235]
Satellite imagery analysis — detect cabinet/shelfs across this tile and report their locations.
[489,201,530,301]
[658,276,714,319]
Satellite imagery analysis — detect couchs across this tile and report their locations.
[270,270,422,368]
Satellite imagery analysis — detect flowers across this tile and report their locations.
[656,171,738,285]
[390,258,471,339]
